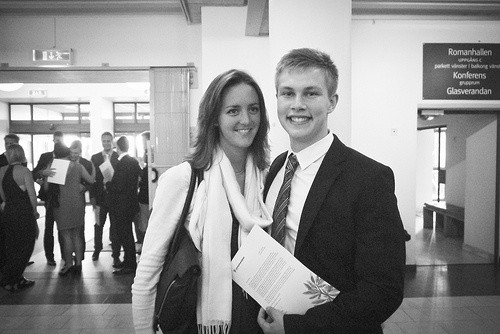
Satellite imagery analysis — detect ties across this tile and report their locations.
[271,154,299,248]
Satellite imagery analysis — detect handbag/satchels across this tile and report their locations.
[152,159,204,334]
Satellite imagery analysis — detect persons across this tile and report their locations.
[134,130,150,255]
[257,48,407,334]
[43,143,96,276]
[0,143,40,292]
[0,133,35,265]
[131,69,273,334]
[103,136,140,275]
[89,132,121,265]
[35,139,93,261]
[32,131,76,266]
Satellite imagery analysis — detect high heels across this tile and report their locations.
[59,265,82,277]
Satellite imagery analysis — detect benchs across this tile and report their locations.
[423,200,464,237]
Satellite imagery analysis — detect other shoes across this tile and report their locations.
[47,259,56,266]
[92,251,99,260]
[112,262,136,274]
[114,257,120,264]
[5,276,35,291]
